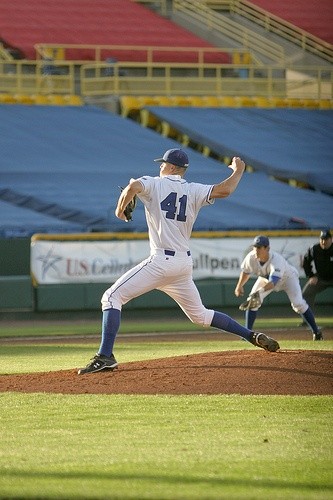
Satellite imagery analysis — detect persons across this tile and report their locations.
[78,149,281,375]
[235,234,323,342]
[297,230,333,329]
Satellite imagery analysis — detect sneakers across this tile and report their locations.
[313,330,323,341]
[250,332,281,352]
[78,353,118,375]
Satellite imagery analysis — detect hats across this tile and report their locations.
[251,236,269,246]
[320,230,332,239]
[154,149,190,167]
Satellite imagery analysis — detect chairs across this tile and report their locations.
[0,0,235,64]
[244,0,333,47]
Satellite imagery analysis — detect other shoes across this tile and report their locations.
[297,322,309,329]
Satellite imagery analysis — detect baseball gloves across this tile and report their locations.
[120,186,137,221]
[238,291,261,311]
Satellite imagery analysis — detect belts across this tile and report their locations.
[165,250,191,256]
